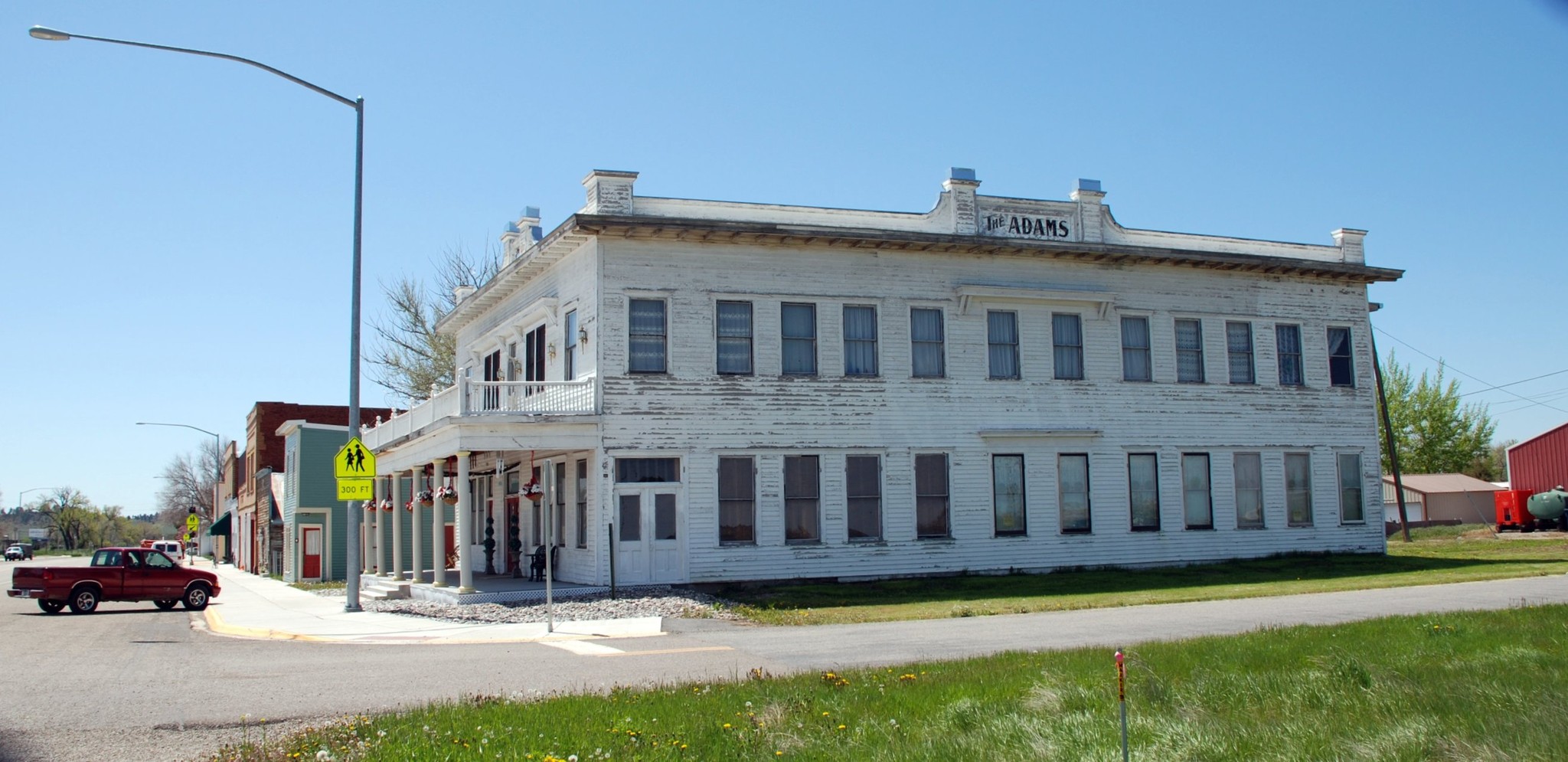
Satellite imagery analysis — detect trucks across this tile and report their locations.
[4,543,32,561]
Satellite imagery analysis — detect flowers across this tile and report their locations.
[361,486,457,510]
[518,484,542,497]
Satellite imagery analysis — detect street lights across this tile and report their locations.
[136,421,219,565]
[153,476,202,556]
[1369,300,1411,542]
[29,25,364,612]
[19,487,64,507]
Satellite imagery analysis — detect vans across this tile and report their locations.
[140,538,185,565]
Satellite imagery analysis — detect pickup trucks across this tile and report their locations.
[6,547,221,615]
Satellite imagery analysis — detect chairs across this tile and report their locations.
[524,545,556,582]
[115,555,133,566]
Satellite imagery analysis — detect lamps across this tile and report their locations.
[515,361,521,380]
[548,344,555,365]
[579,325,587,354]
[497,369,504,381]
